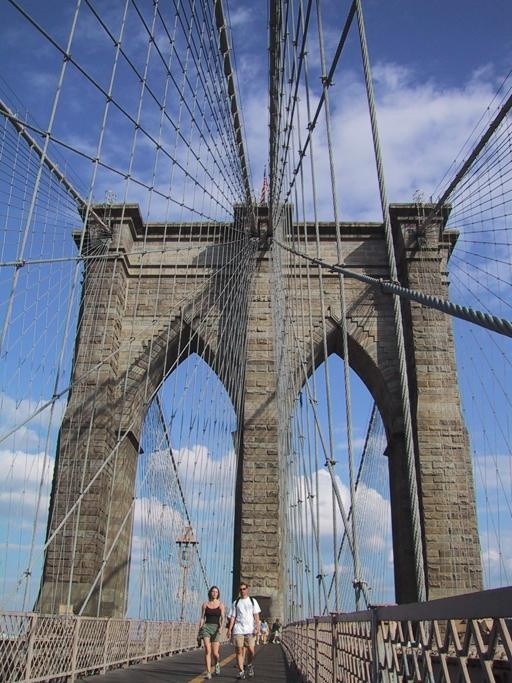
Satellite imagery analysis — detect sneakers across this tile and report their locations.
[247,665,254,676]
[236,670,245,680]
[204,672,212,680]
[215,664,221,675]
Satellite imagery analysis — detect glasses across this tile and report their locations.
[240,588,246,591]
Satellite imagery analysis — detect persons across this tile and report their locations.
[197,586,225,679]
[235,618,281,670]
[227,582,261,680]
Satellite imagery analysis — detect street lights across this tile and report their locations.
[176,526,199,623]
